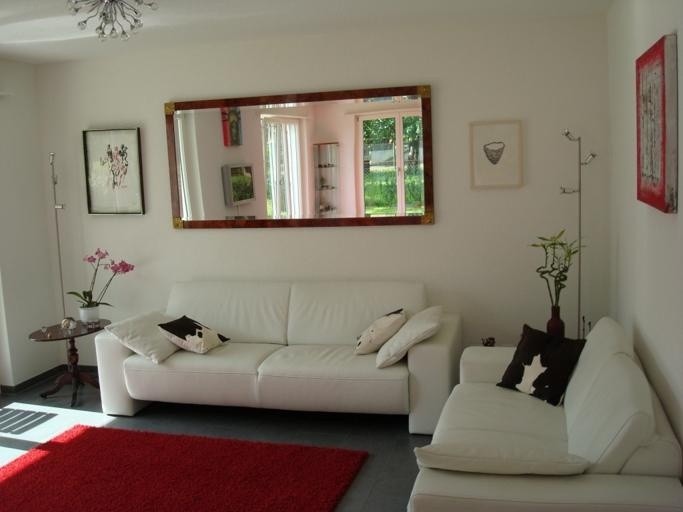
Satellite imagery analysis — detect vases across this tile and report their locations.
[67,248,134,306]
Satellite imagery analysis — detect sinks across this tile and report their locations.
[98,282,460,437]
[408,316,680,511]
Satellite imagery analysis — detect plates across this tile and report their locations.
[313,142,338,218]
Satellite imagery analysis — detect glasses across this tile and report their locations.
[65,0,157,43]
[555,128,599,341]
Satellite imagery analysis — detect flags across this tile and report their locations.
[79,306,99,324]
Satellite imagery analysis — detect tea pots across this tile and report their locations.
[164,86,434,227]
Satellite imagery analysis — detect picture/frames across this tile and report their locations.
[28,320,111,406]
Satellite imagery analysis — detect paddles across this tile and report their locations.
[0,419,368,512]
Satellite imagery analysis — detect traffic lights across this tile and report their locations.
[412,437,591,479]
[354,308,406,355]
[158,314,230,355]
[375,305,442,369]
[105,308,185,362]
[494,322,587,408]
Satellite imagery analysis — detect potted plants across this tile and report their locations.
[80,126,144,215]
[468,119,525,190]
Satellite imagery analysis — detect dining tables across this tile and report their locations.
[531,229,584,336]
